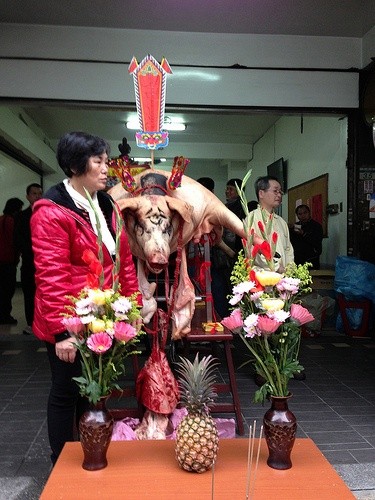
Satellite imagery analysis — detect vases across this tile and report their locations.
[263,393,296,470]
[80,394,114,471]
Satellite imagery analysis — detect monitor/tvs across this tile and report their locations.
[267,157,284,190]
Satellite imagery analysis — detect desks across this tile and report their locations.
[38,437,358,500]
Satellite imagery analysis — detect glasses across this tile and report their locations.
[262,189,284,195]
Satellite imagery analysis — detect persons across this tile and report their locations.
[210,177,253,318]
[286,204,323,269]
[196,176,215,192]
[17,184,46,336]
[28,131,145,466]
[0,196,25,328]
[239,176,308,388]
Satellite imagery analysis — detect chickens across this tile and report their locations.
[137,246,196,341]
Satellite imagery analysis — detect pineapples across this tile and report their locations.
[171,352,221,473]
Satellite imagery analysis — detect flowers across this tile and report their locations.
[60,186,145,405]
[221,170,315,402]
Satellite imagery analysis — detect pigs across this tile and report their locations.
[105,168,281,276]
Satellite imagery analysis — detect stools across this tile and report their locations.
[181,328,246,436]
[95,340,145,419]
[302,296,326,338]
[335,294,370,336]
